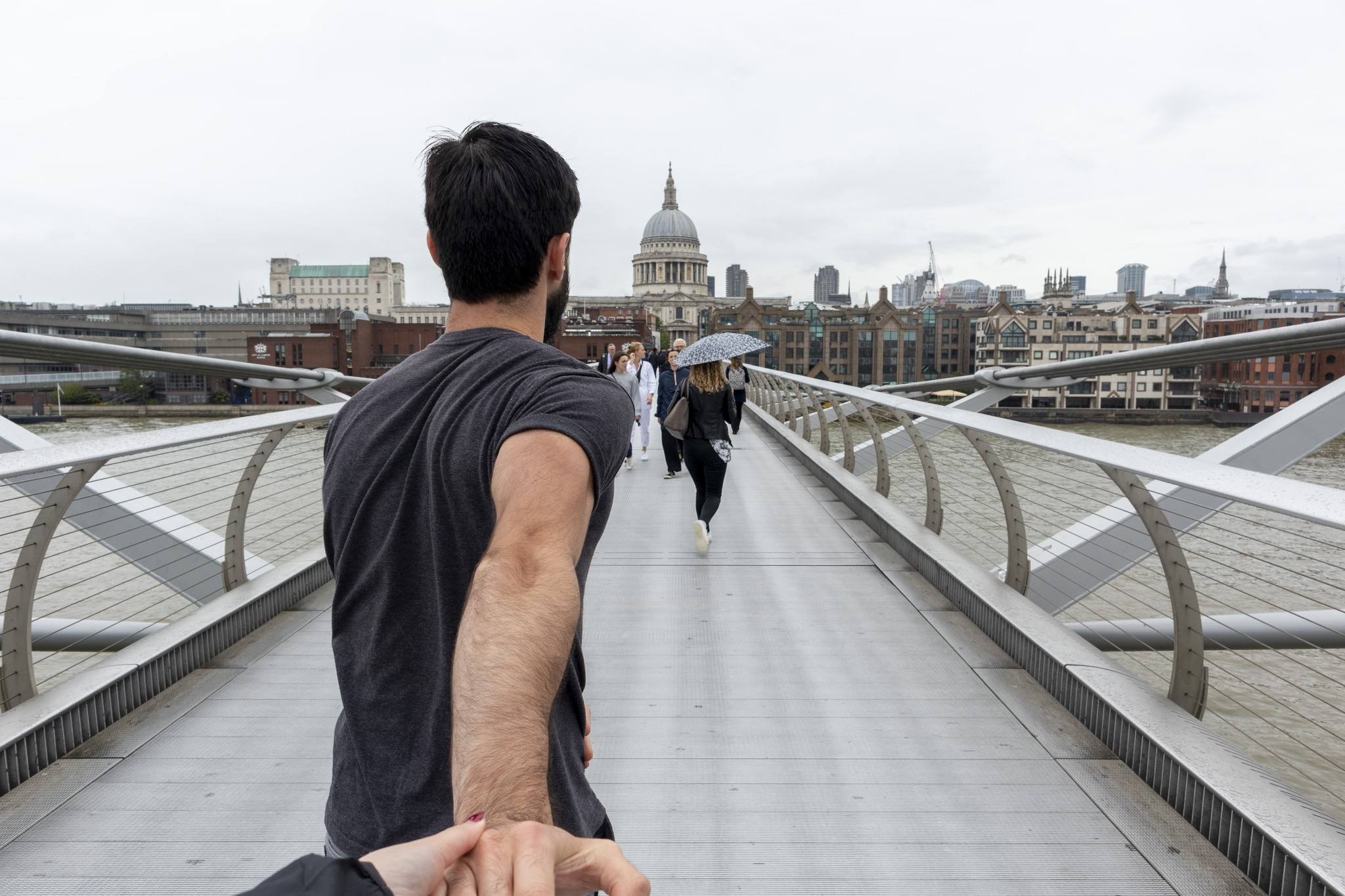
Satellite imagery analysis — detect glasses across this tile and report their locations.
[674,346,686,349]
[608,349,615,351]
[667,348,678,353]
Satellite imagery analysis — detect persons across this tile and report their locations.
[726,355,750,434]
[673,338,686,462]
[606,352,641,477]
[667,359,737,555]
[628,340,672,384]
[655,350,691,479]
[231,812,485,896]
[625,342,656,463]
[324,119,649,896]
[597,343,616,375]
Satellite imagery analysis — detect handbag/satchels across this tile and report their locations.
[664,397,690,440]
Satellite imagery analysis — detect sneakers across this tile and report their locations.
[627,453,633,470]
[664,470,675,479]
[692,520,712,555]
[641,451,648,461]
[624,459,627,463]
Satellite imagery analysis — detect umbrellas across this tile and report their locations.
[745,348,768,354]
[673,333,772,369]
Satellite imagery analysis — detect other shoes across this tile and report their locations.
[733,431,737,434]
[678,453,684,463]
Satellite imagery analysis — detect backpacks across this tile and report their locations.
[728,366,746,390]
